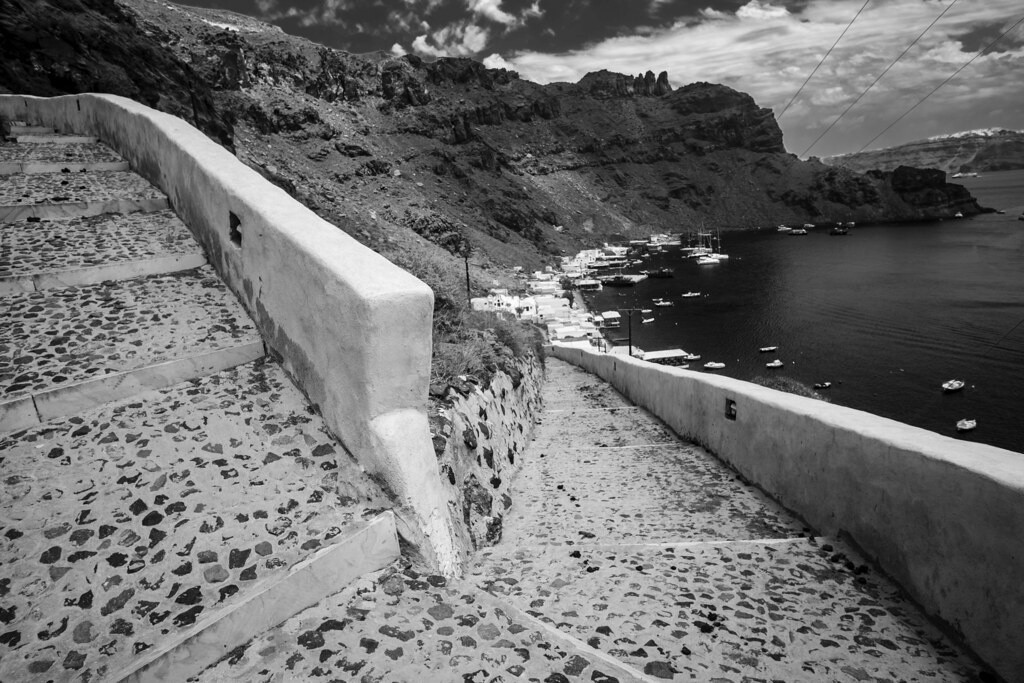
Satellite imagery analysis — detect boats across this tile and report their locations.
[696,253,729,265]
[642,309,653,314]
[830,221,856,235]
[681,292,700,297]
[952,172,978,178]
[652,298,663,301]
[814,381,831,389]
[578,233,713,291]
[704,361,726,369]
[765,361,784,368]
[642,348,690,369]
[942,378,965,391]
[683,352,700,361]
[759,346,778,352]
[641,318,655,324]
[593,310,622,329]
[956,418,977,430]
[654,301,673,307]
[777,223,815,235]
